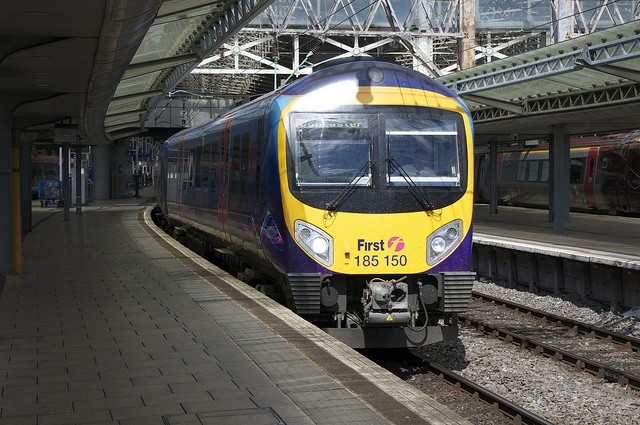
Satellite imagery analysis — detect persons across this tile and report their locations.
[392,148,436,177]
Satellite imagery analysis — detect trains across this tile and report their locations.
[154,61,476,348]
[475,128,640,215]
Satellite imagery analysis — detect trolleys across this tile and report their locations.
[37,179,65,208]
